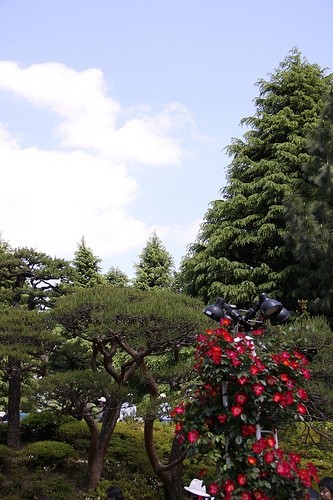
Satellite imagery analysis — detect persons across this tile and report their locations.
[316,477,333,500]
[1,392,181,426]
[183,478,210,500]
[102,486,124,500]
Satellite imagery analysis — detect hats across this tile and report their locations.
[184,478,210,497]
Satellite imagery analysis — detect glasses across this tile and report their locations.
[107,494,115,498]
[184,491,198,498]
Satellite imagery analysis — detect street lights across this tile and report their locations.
[203,292,291,500]
